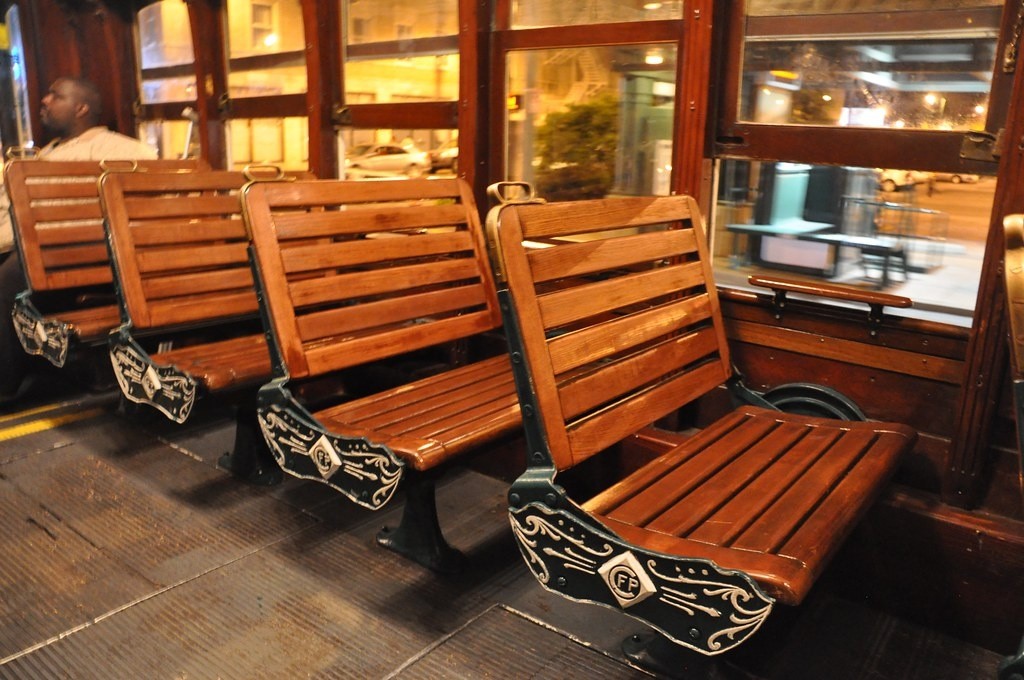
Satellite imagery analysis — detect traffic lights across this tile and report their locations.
[508,94,522,112]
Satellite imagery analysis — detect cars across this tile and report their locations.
[344,143,459,174]
[878,169,978,192]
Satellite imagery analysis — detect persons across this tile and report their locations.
[0,76,158,414]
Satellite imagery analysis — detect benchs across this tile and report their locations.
[486,181,921,679]
[4,147,216,421]
[96,157,354,486]
[239,165,524,580]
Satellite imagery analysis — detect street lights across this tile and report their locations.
[179,78,197,159]
[247,34,278,163]
